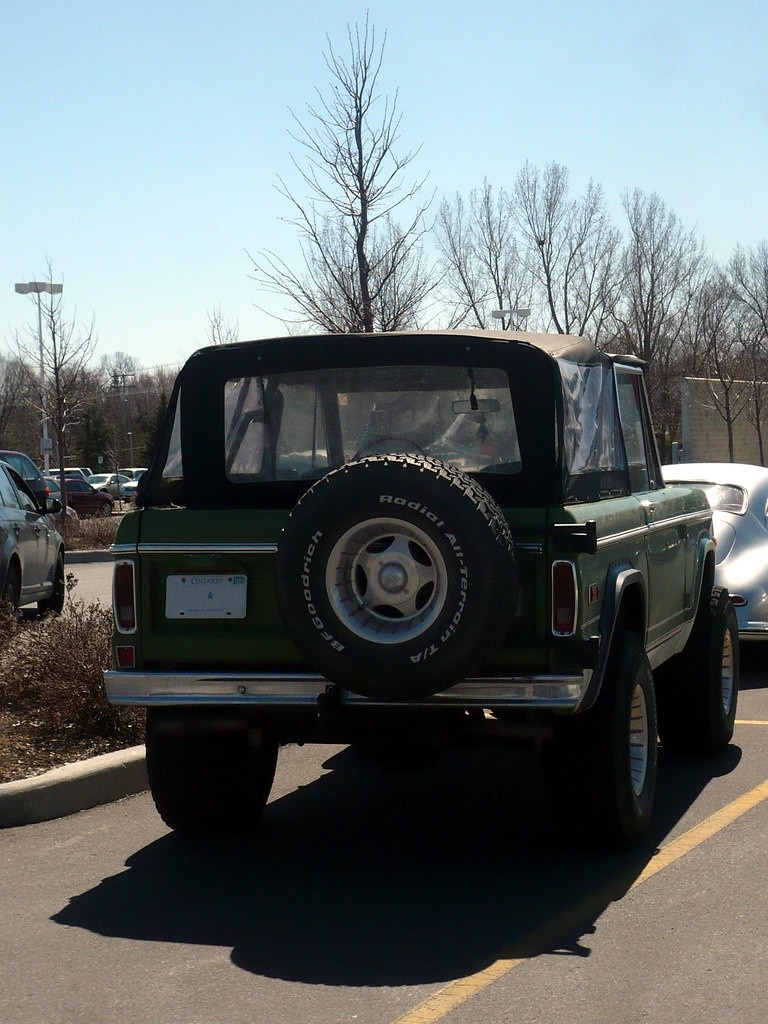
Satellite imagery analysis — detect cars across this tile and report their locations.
[660,463,768,648]
[0,460,64,618]
[43,477,115,519]
[44,467,148,501]
[0,450,48,510]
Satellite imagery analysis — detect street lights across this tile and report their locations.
[16,283,62,478]
[492,308,531,332]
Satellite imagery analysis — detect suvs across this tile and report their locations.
[101,331,741,837]
